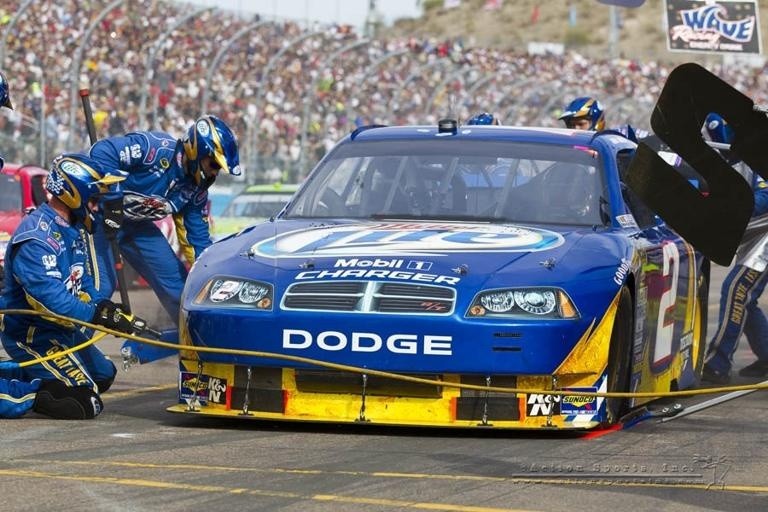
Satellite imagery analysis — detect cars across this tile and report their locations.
[0,162,194,293]
[164,119,710,440]
[208,182,301,243]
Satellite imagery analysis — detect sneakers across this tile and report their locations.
[740,361,767,377]
[701,363,733,385]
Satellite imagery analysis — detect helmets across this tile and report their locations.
[464,112,502,125]
[46,153,130,234]
[558,96,605,132]
[182,115,241,192]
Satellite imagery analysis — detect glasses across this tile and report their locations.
[209,157,221,170]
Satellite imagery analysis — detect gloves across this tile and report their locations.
[104,198,123,232]
[92,299,134,338]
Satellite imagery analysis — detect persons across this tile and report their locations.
[0,0,768,187]
[538,160,594,223]
[702,112,767,385]
[87,115,244,329]
[557,98,652,141]
[0,153,140,420]
